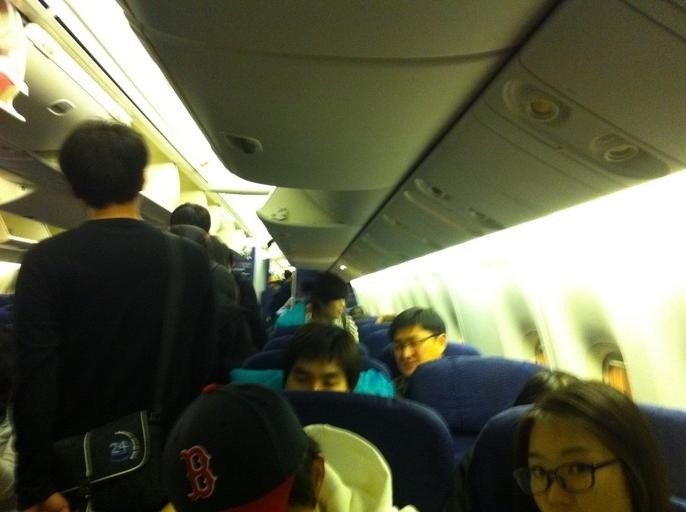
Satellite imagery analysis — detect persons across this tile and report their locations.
[388,306,449,401]
[456,371,673,512]
[2,121,421,512]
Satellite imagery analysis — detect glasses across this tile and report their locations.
[511,456,621,496]
[391,331,444,352]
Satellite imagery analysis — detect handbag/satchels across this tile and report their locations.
[52,408,169,511]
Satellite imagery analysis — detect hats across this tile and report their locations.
[268,276,278,284]
[285,270,292,278]
[310,274,346,301]
[162,383,308,512]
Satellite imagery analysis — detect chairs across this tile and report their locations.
[199,296,685,509]
